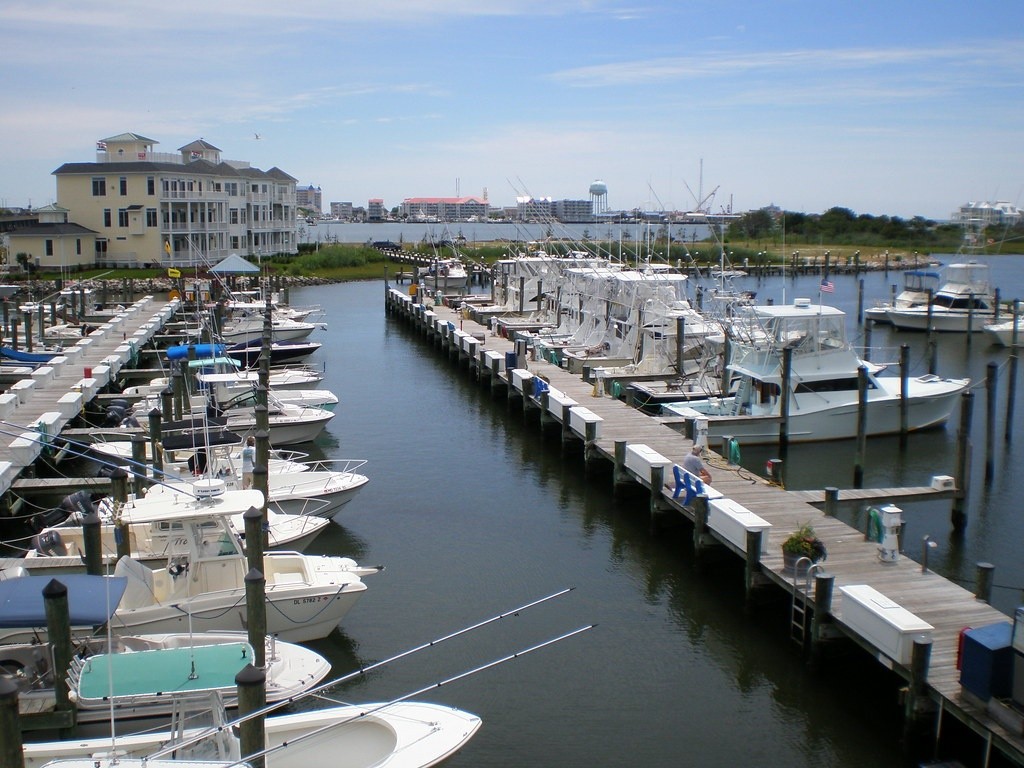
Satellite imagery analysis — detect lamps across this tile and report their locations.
[533,335,540,346]
[879,502,903,528]
[694,413,709,431]
[593,364,604,380]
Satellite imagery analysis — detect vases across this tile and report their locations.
[784,551,818,573]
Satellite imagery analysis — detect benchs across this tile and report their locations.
[420,303,426,311]
[672,464,725,506]
[447,320,456,331]
[531,375,559,403]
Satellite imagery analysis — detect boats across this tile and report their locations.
[0,157,971,768]
[882,259,1024,333]
[863,269,947,326]
[982,318,1024,349]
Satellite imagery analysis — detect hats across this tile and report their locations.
[693,445,702,454]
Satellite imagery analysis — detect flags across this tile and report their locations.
[165,241,170,254]
[821,279,835,293]
[168,268,181,278]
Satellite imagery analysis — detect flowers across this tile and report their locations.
[780,517,829,562]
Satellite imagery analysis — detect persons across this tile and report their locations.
[683,444,712,485]
